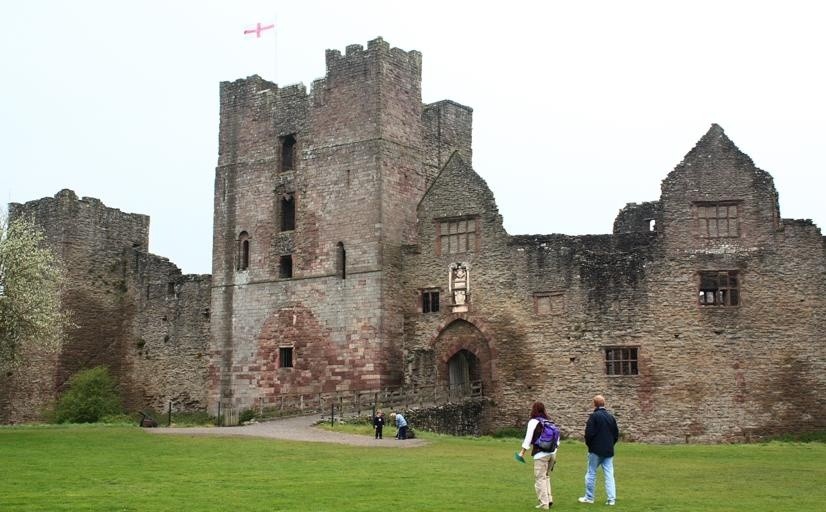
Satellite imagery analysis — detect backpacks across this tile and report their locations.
[534,416,560,453]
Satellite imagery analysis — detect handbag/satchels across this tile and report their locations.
[405,430,415,439]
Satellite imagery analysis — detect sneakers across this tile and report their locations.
[604,500,615,506]
[535,502,553,510]
[577,496,594,504]
[374,436,404,440]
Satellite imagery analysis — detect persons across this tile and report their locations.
[372,410,385,439]
[577,393,621,505]
[518,401,561,510]
[389,412,408,440]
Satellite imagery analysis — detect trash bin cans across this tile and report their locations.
[224,407,239,426]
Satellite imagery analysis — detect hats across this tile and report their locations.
[376,411,396,419]
